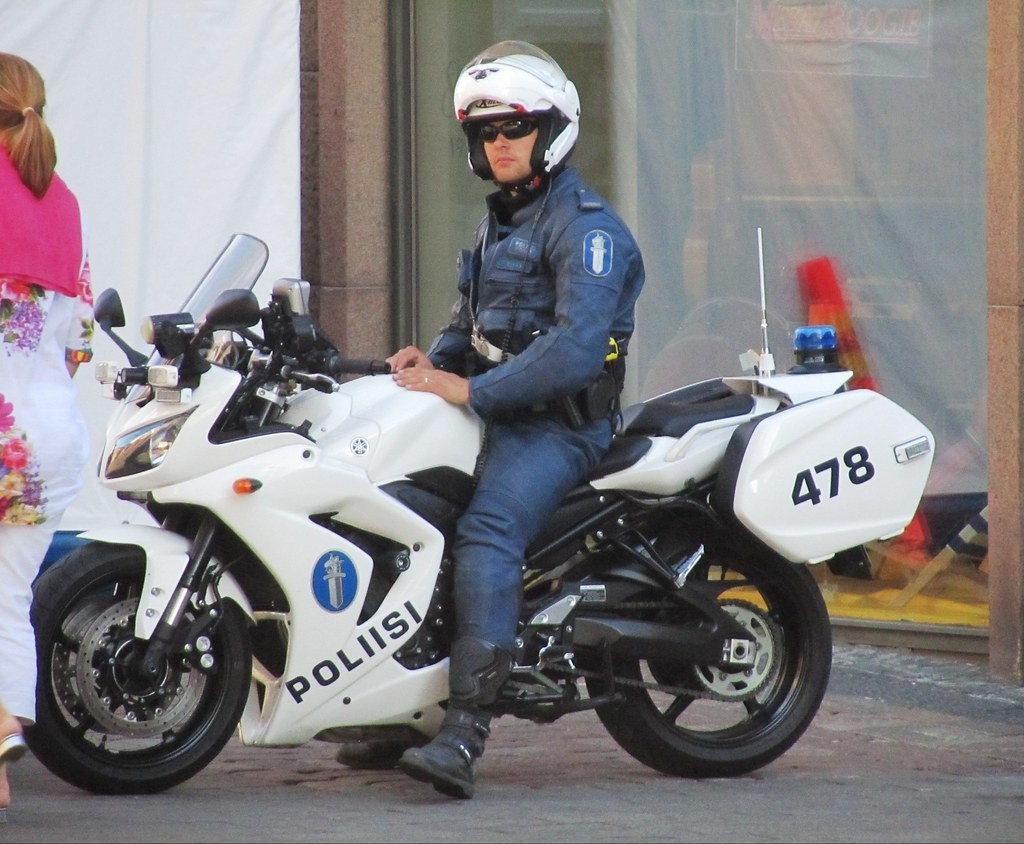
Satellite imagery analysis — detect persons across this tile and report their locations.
[334,39,646,799]
[0,54,94,817]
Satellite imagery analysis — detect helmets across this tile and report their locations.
[454,40,580,181]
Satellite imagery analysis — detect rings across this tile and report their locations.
[425,378,428,382]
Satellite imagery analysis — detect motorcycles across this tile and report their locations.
[21,232,937,794]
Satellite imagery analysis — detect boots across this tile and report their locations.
[399,635,514,801]
[336,737,399,770]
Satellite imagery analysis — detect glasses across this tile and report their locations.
[480,119,539,144]
